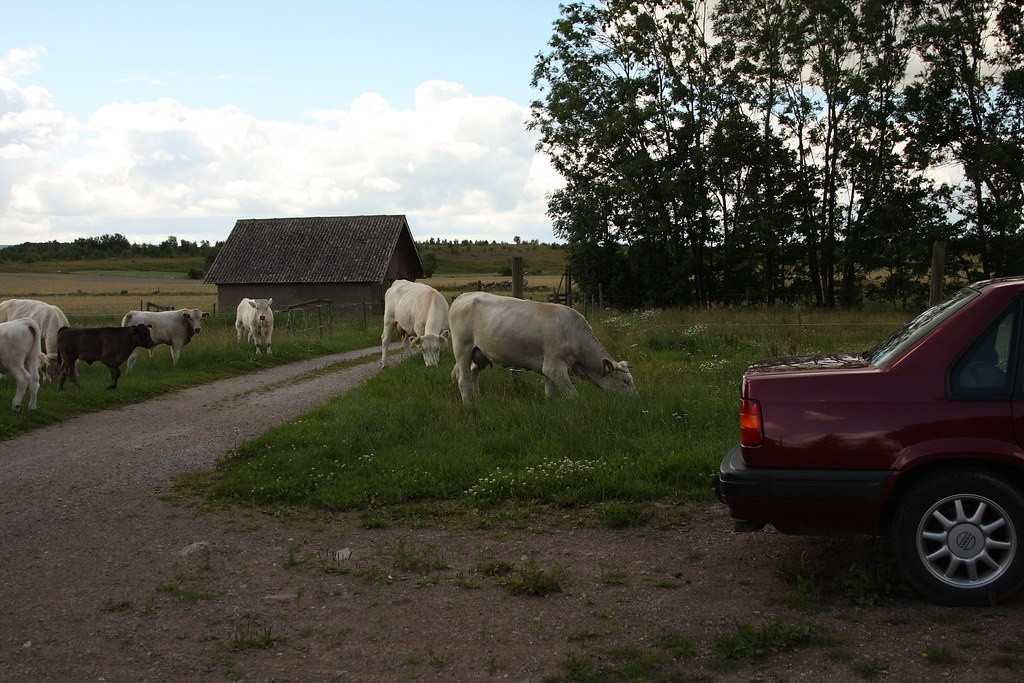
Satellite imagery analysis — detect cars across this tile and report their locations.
[713,279,1023,607]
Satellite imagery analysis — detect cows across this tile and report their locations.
[381,279,449,371]
[121,308,211,374]
[448,290,640,408]
[56,323,155,392]
[0,299,80,413]
[236,297,274,356]
